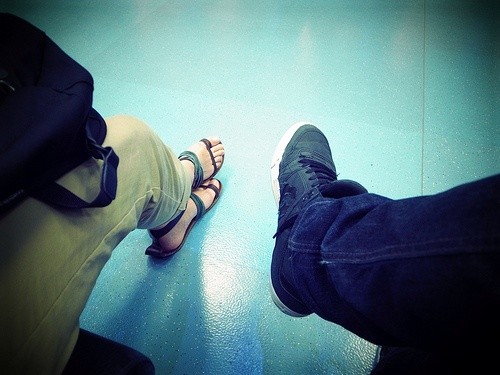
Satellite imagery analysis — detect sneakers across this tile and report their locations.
[268,121,338,319]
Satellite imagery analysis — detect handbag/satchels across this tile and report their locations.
[0,10,120,209]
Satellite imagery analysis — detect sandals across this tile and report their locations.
[177,138,226,191]
[144,177,222,258]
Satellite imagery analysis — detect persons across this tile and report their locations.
[0,116,225,375]
[266,121,500,375]
